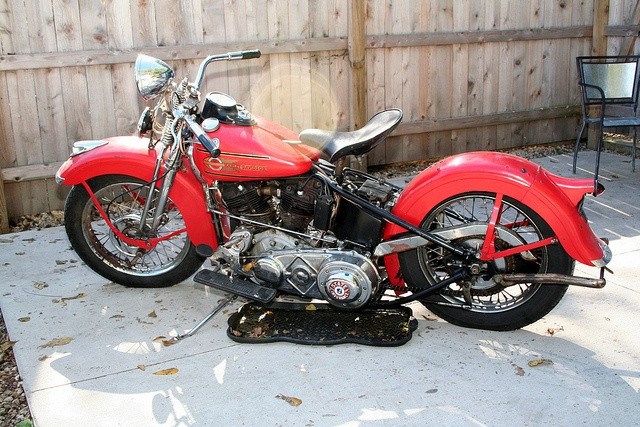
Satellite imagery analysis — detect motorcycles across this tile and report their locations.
[55,49,614,340]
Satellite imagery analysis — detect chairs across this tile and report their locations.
[572,55,639,180]
